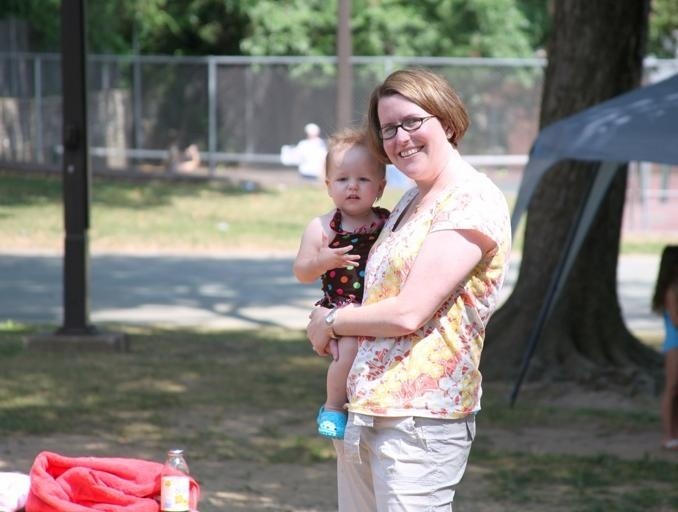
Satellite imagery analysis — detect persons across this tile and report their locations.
[166,137,199,173]
[279,124,326,181]
[305,69,510,512]
[291,128,394,439]
[651,244,676,449]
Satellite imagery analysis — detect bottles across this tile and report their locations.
[160,449,191,512]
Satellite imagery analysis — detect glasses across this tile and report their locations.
[378,116,436,140]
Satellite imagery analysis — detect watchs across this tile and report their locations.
[324,307,340,339]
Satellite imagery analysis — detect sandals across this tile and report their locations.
[317,406,347,439]
